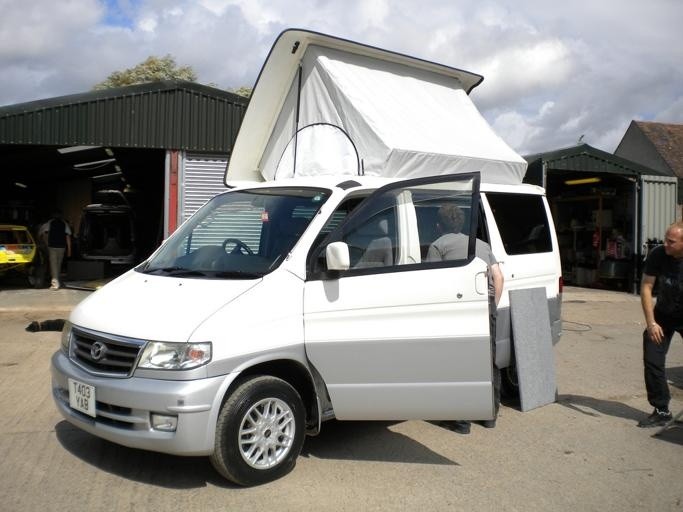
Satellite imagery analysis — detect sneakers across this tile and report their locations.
[639,406,673,428]
[48,279,61,291]
[439,420,497,435]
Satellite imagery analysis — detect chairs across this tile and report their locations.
[271,216,308,260]
[346,214,394,270]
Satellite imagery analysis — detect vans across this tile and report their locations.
[47,26,570,488]
[79,190,134,267]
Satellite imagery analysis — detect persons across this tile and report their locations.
[422,204,505,432]
[41,214,70,290]
[634,220,683,430]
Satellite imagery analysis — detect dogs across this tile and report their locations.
[25,319,65,332]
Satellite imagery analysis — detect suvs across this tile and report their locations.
[0,225,40,285]
